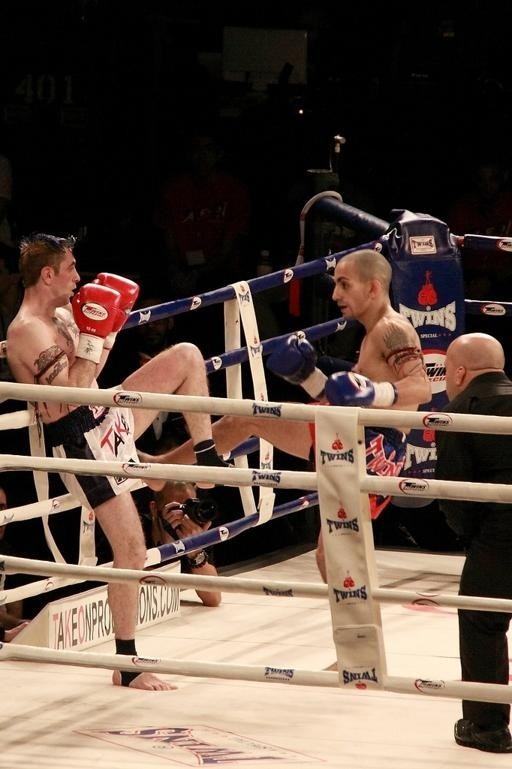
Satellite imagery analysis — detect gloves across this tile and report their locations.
[323,370,398,408]
[263,329,330,399]
[71,270,143,366]
